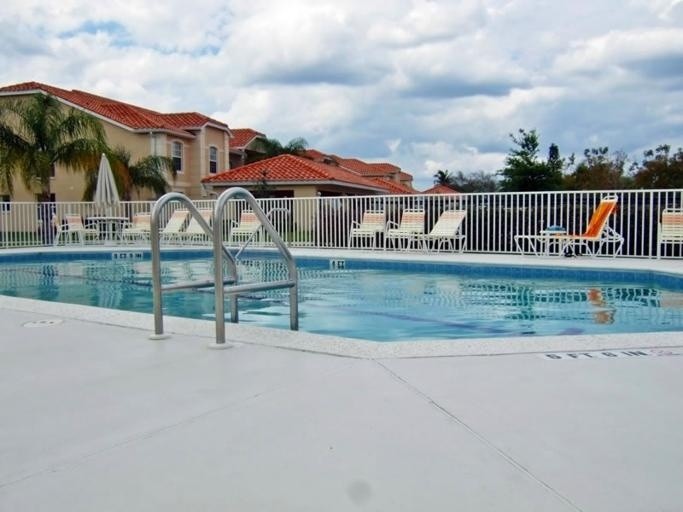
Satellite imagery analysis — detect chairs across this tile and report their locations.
[348,206,468,253]
[51,208,264,248]
[513,195,625,254]
[656,210,683,258]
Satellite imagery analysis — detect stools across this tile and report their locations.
[539,230,568,256]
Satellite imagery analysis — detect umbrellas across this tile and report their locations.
[95,153,120,208]
